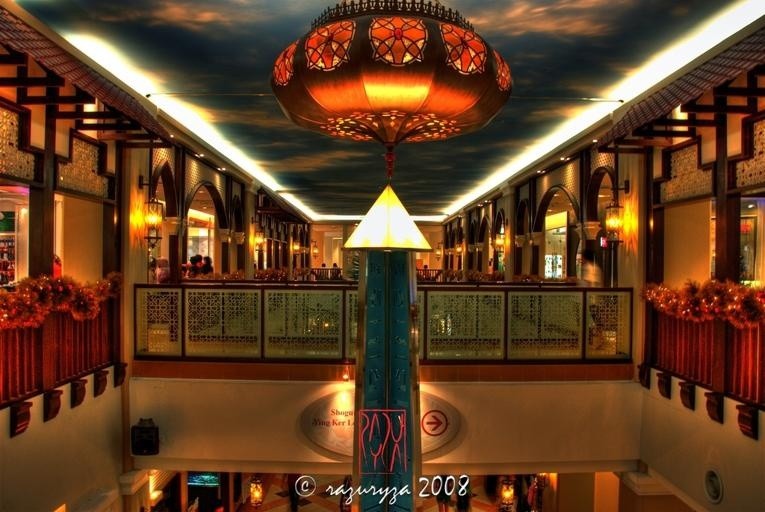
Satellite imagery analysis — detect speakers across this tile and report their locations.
[131,425,159,455]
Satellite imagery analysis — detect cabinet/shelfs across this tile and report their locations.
[0,191,28,290]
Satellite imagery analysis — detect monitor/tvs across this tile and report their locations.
[187,470,222,487]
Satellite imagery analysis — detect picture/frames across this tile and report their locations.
[709,213,759,282]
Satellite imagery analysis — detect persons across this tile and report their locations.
[455,476,473,512]
[149,255,215,279]
[423,265,431,280]
[434,474,454,512]
[740,257,750,279]
[318,263,330,280]
[280,474,303,511]
[329,263,342,279]
[254,263,259,278]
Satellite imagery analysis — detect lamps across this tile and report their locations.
[433,216,509,260]
[601,177,630,244]
[249,473,264,509]
[136,172,164,250]
[261,1,513,260]
[254,230,319,260]
[497,475,516,510]
